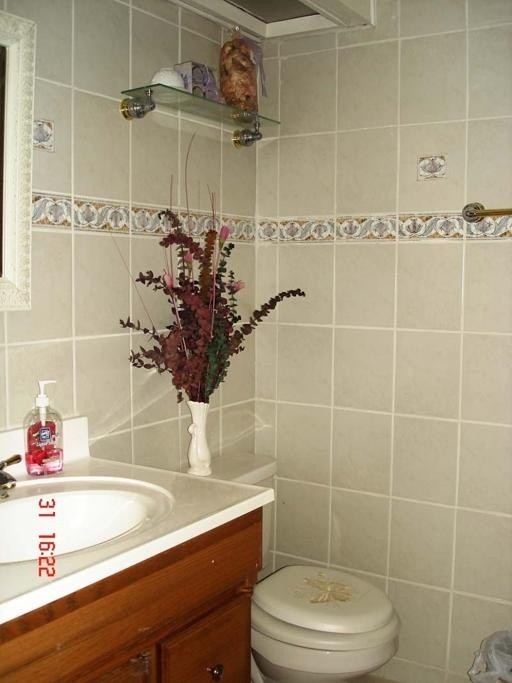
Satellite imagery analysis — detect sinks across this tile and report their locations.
[0,475,178,568]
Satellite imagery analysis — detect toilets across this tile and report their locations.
[210,447,402,683]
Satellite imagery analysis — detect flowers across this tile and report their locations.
[104,133,305,400]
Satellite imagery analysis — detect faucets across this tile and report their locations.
[0,454,22,501]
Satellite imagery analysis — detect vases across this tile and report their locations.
[186,401,211,476]
